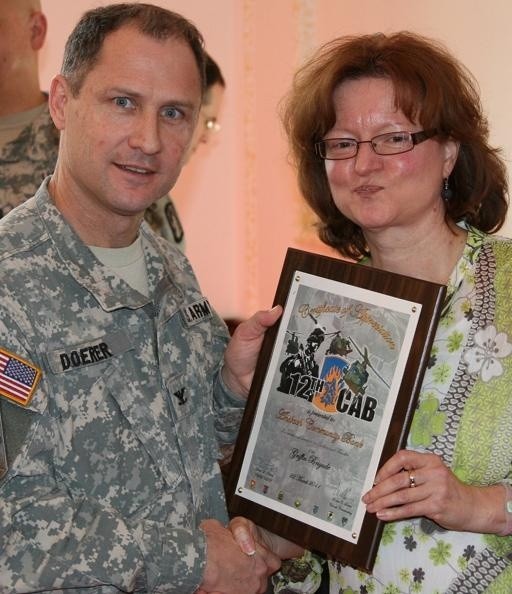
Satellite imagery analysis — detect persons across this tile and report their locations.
[1,1,283,593]
[232,31,511,593]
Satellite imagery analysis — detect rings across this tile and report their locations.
[409,469,416,487]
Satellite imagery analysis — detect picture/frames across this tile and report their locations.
[224,247,447,575]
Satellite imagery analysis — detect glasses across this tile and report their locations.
[314,128,438,160]
